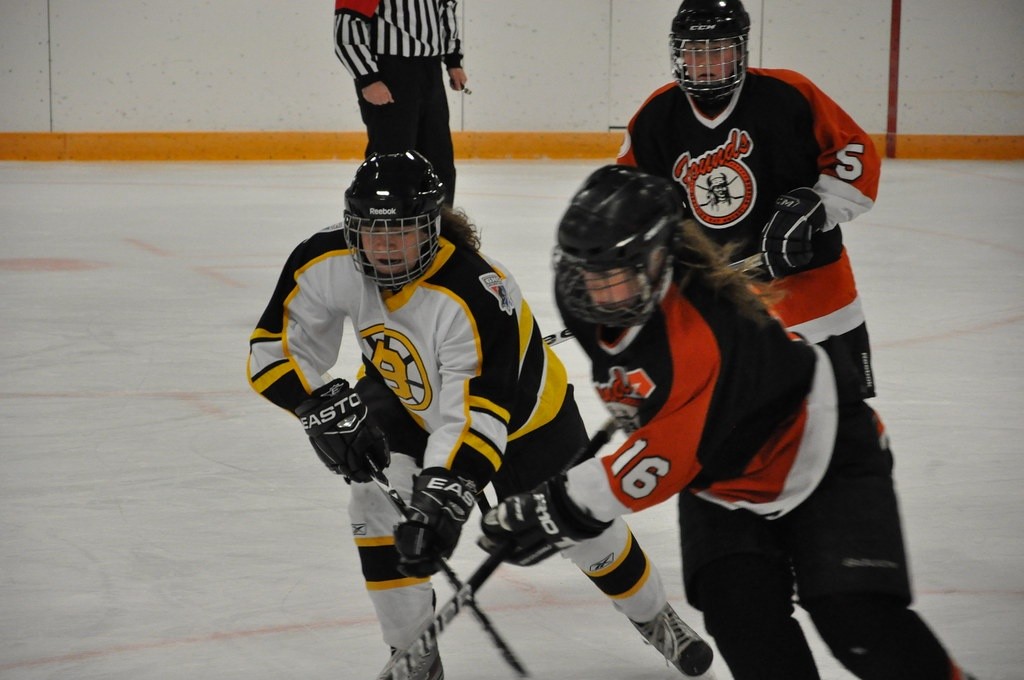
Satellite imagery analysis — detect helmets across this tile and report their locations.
[668,0,750,103]
[555,164,679,330]
[345,150,443,288]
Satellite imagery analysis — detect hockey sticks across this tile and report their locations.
[374,416,618,680]
[371,470,530,680]
[541,247,766,348]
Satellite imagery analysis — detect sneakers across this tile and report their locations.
[382,639,444,680]
[629,600,714,680]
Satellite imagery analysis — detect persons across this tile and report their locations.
[616,0,881,408]
[247,150,714,680]
[333,0,467,208]
[481,163,979,680]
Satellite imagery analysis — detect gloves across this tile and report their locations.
[394,473,475,577]
[294,379,389,485]
[757,188,828,278]
[477,471,616,566]
